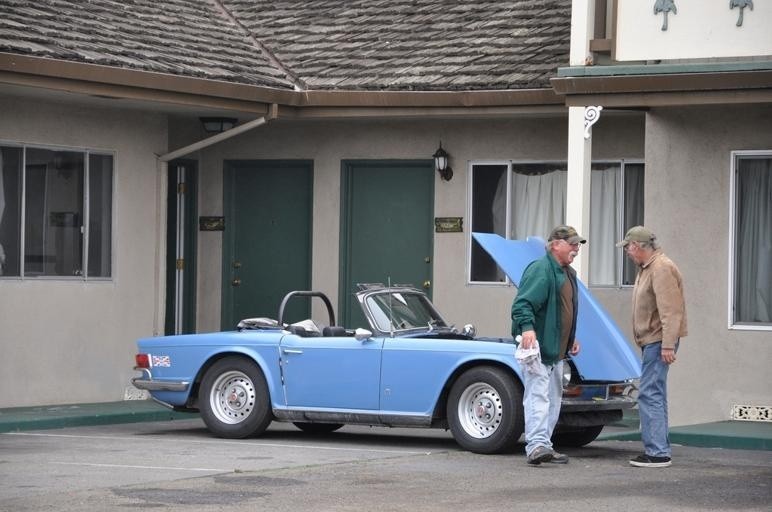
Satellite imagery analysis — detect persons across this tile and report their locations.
[614,225,688,467]
[509,224,589,464]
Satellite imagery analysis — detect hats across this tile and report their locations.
[548,225,586,245]
[616,226,656,248]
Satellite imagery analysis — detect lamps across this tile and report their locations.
[431,139,454,181]
[199,118,240,134]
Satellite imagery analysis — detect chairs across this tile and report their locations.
[287,324,383,336]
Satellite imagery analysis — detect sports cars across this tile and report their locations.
[127,232,642,455]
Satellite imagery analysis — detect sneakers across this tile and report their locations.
[629,454,672,467]
[527,446,553,464]
[541,450,568,463]
